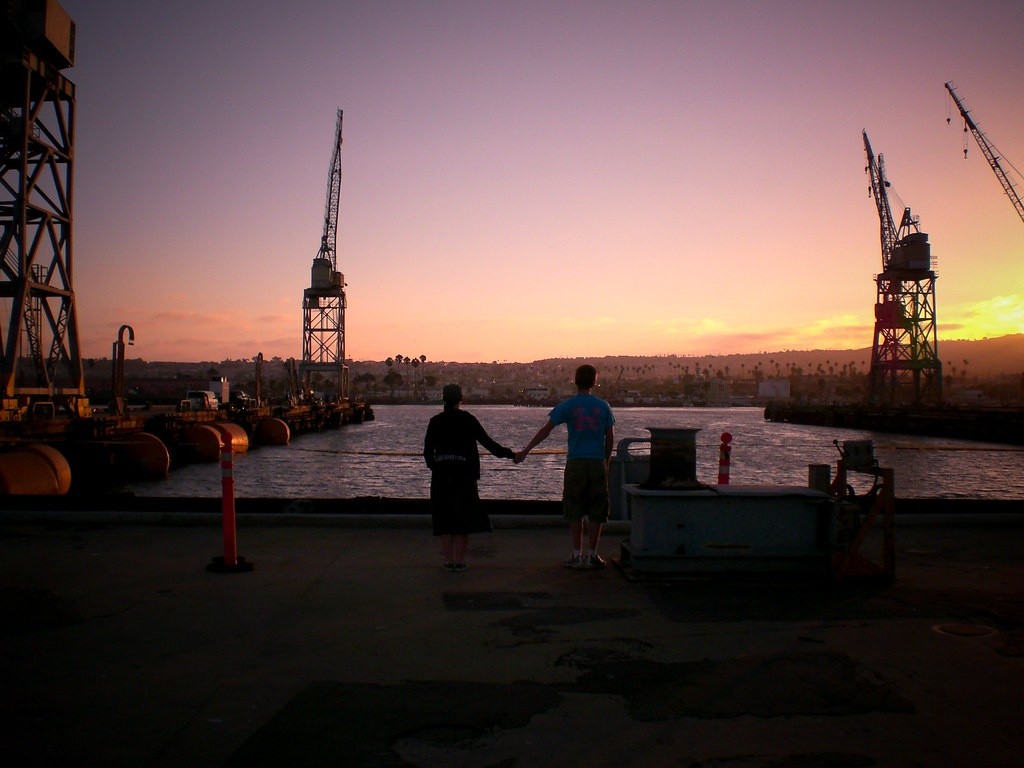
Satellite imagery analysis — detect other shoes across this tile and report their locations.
[565,552,585,569]
[587,553,607,567]
[442,563,454,571]
[454,563,468,571]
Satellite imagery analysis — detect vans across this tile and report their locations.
[186,391,218,410]
[229,390,249,402]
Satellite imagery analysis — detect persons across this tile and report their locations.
[513,365,615,570]
[423,384,526,572]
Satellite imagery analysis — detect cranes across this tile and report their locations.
[944,80,1024,221]
[298,108,349,401]
[862,127,944,413]
